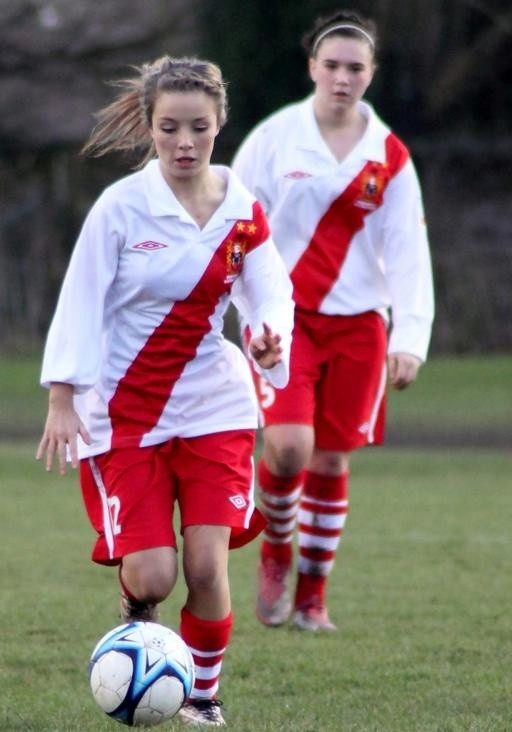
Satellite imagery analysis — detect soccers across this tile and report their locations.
[89,619,196,724]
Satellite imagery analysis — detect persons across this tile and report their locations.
[232,12,437,633]
[35,51,297,728]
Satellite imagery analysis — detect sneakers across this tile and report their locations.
[294,607,336,635]
[258,561,293,627]
[179,702,225,728]
